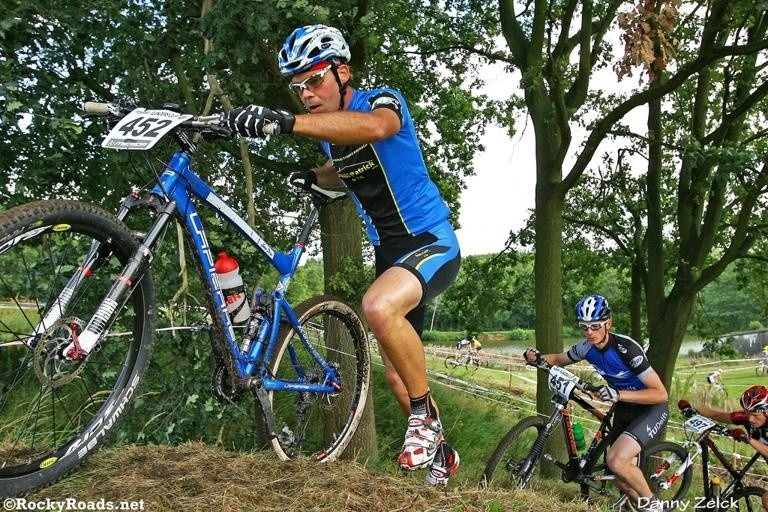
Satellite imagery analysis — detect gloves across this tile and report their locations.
[229,103,299,139]
[286,168,320,192]
[597,386,621,404]
[526,348,542,363]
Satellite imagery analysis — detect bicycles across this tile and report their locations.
[755,356,767,378]
[633,399,768,512]
[706,382,729,400]
[0,96,375,496]
[443,341,480,372]
[474,346,647,511]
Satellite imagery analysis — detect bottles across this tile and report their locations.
[214,250,251,325]
[711,475,721,498]
[571,421,586,451]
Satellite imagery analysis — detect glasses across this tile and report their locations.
[288,63,331,97]
[578,319,609,331]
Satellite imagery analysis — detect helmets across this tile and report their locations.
[279,23,351,78]
[575,294,608,321]
[740,385,768,412]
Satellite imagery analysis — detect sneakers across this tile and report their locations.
[640,498,663,512]
[425,445,459,487]
[397,397,444,472]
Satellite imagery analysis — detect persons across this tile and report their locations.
[467,334,482,359]
[228,25,461,490]
[707,368,724,394]
[523,296,669,510]
[679,385,768,512]
